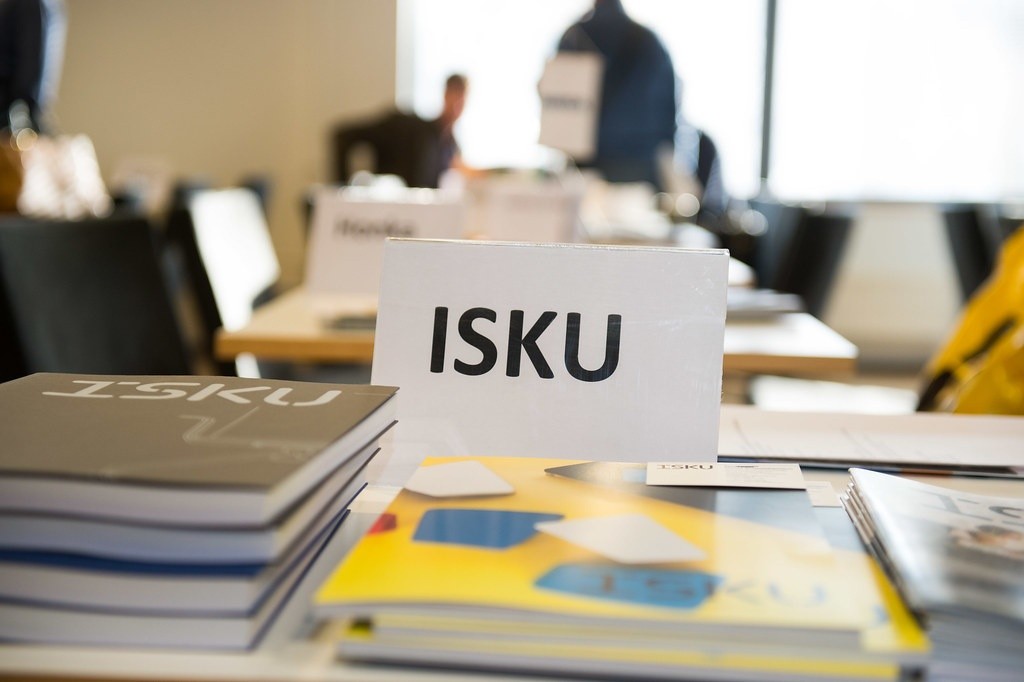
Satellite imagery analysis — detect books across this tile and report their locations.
[2,365,1021,677]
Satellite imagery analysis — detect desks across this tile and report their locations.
[204,251,860,403]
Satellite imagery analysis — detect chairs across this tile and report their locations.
[721,200,853,316]
[0,174,361,383]
[942,209,996,301]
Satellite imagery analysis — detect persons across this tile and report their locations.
[326,73,470,188]
